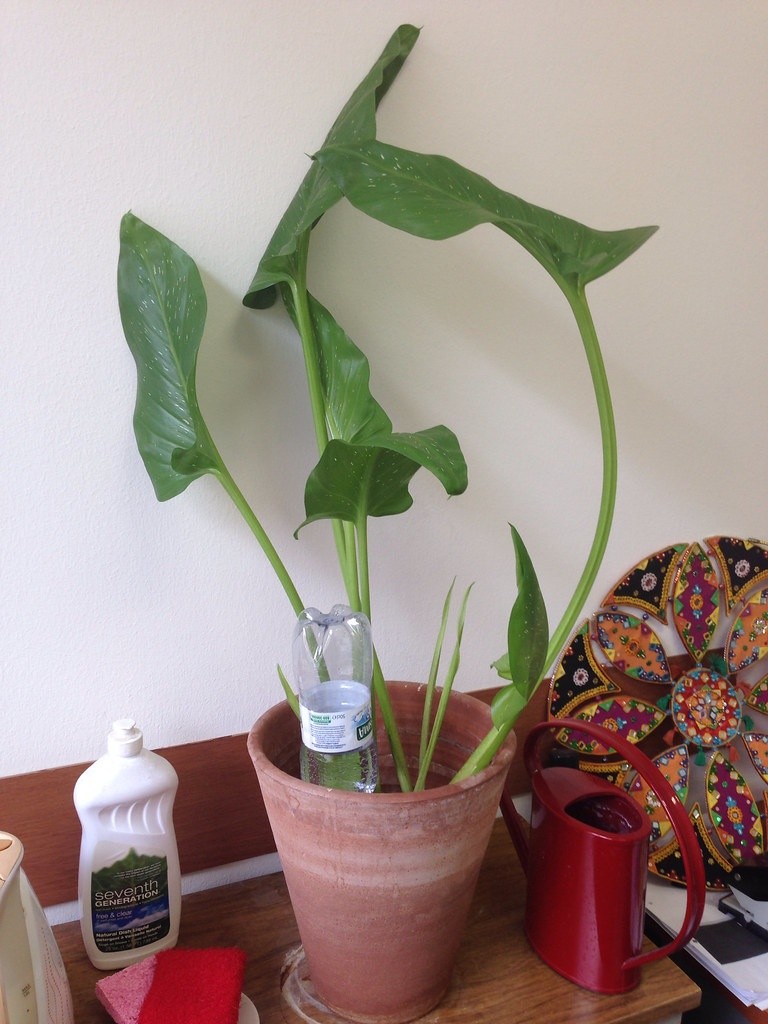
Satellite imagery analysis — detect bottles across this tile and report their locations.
[75,719,182,969]
[294,605,381,792]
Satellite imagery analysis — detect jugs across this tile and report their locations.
[499,718,705,996]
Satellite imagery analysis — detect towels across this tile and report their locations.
[134,945,249,1024]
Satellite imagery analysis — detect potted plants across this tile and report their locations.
[116,24,663,1024]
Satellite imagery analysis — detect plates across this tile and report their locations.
[238,992,260,1024]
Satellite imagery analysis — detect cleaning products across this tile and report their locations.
[72,716,183,972]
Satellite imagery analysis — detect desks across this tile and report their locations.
[48,813,703,1024]
